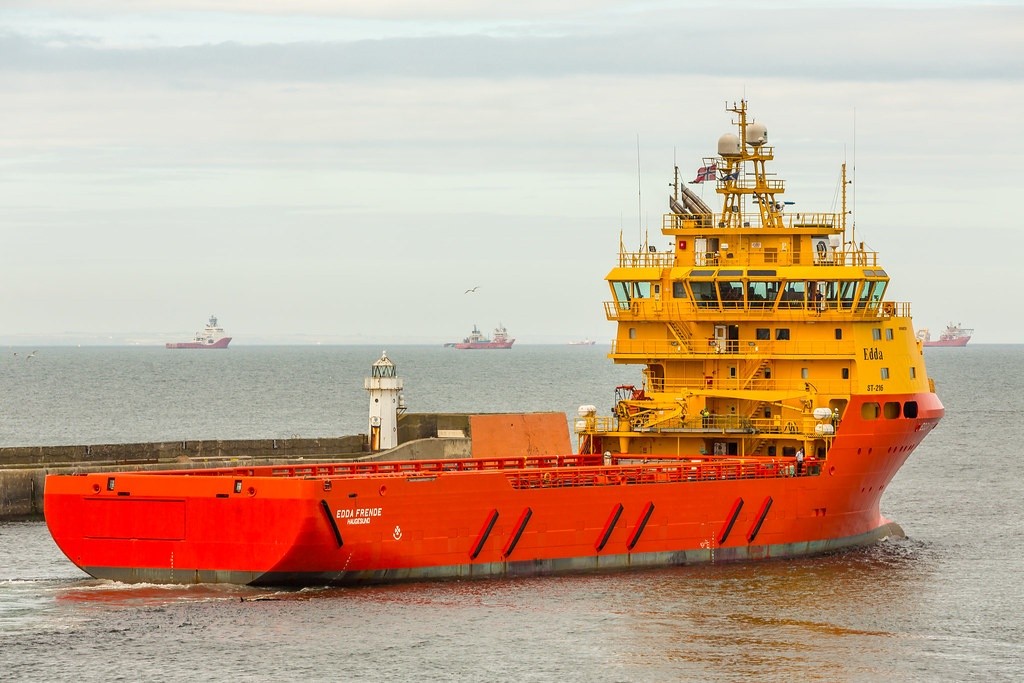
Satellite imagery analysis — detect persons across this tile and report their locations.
[814,290,824,317]
[700,408,709,428]
[715,445,723,455]
[832,408,841,435]
[794,447,804,477]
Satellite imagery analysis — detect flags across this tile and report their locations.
[688,163,717,184]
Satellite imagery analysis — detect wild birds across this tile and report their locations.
[464,285,481,296]
[13,349,40,360]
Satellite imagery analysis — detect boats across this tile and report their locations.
[915,321,975,348]
[42,96,946,598]
[164,315,233,350]
[443,325,516,350]
